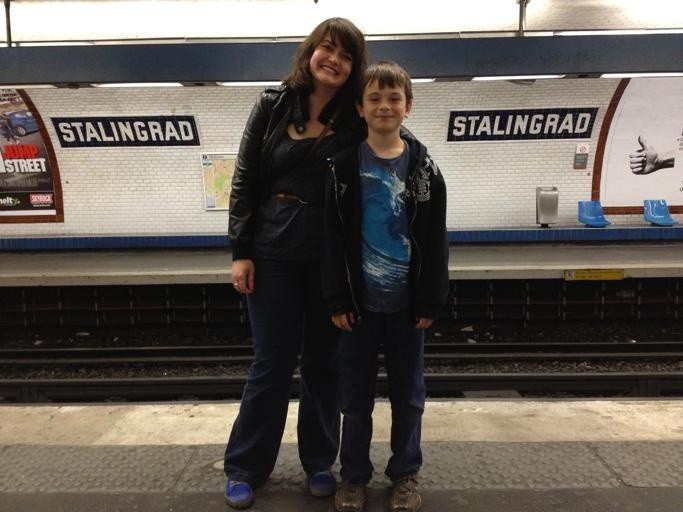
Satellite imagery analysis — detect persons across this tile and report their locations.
[223,16,391,510]
[627,135,674,175]
[327,60,451,511]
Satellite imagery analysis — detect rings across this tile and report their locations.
[234,283,238,286]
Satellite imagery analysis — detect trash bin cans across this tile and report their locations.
[536,186,558,224]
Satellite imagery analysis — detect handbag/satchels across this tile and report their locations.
[250,193,309,256]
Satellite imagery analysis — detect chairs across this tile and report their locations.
[577,200,611,229]
[643,198,677,228]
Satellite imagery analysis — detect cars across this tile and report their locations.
[3,107,41,138]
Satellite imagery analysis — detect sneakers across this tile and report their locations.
[391,484,421,511]
[224,477,252,507]
[308,470,336,496]
[333,479,366,512]
